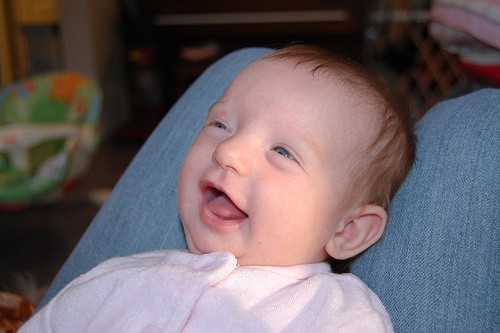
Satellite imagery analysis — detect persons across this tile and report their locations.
[16,43,418,333]
